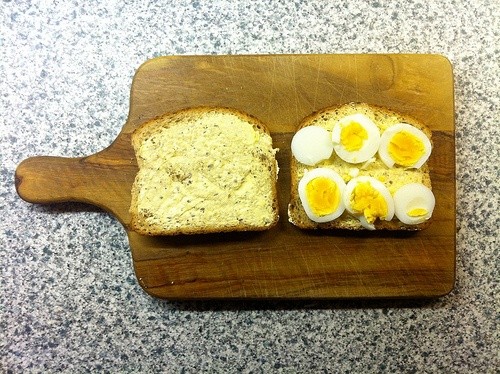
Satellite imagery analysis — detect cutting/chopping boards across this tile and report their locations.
[13,54,456,299]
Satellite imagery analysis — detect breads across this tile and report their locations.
[287,101,434,231]
[128,103,280,235]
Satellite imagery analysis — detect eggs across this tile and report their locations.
[378,122,432,170]
[290,126,333,166]
[343,176,395,230]
[393,183,436,225]
[298,167,347,223]
[332,113,380,163]
